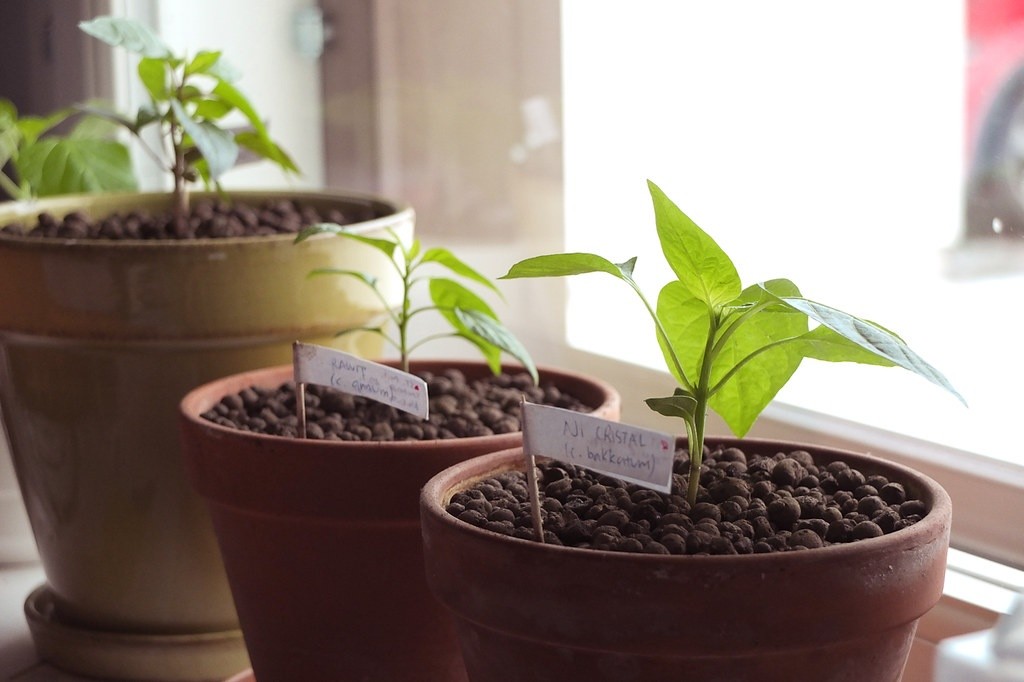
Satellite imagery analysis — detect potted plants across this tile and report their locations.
[414,176,973,680]
[170,217,629,682]
[2,15,414,679]
